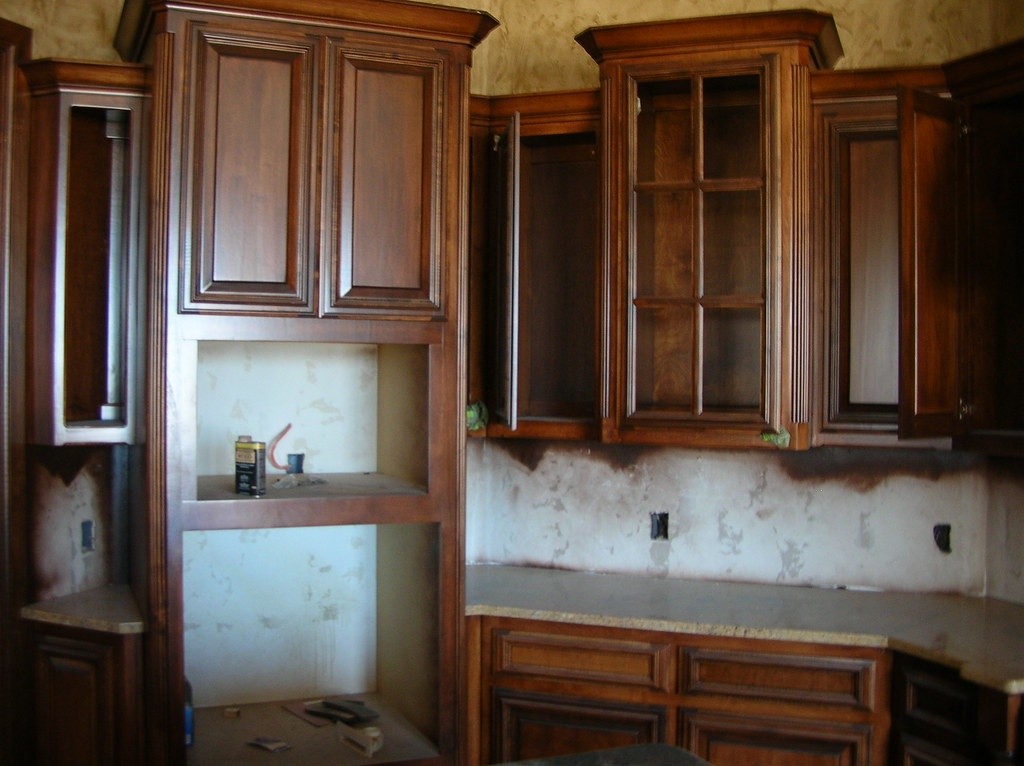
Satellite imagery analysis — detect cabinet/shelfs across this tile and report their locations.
[1,0,1023,765]
